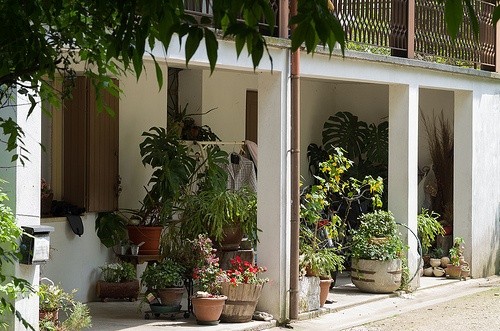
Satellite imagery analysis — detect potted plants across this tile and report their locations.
[419,207,465,277]
[92,129,263,312]
[40,280,94,331]
[297,142,408,308]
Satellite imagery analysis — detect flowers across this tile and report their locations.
[183,229,221,294]
[220,253,262,283]
[98,260,137,281]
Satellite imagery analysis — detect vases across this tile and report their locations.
[192,296,225,321]
[95,281,140,297]
[216,278,265,323]
